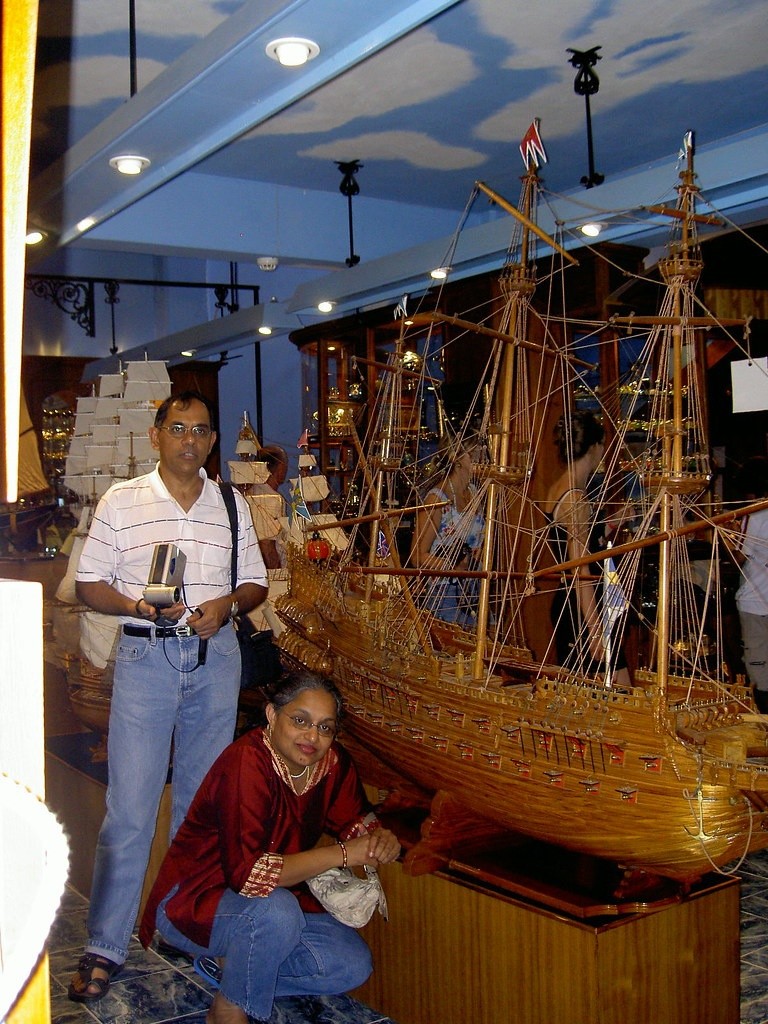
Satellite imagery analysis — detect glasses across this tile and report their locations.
[158,425,212,439]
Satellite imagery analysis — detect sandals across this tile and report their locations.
[67,953,124,1003]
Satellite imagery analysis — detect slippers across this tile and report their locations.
[193,955,224,991]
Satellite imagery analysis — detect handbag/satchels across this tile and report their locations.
[233,615,283,688]
[305,812,391,929]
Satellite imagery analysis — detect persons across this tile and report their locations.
[69,395,269,999]
[139,670,402,1023]
[244,410,768,712]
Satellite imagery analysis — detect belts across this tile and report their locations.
[123,618,231,637]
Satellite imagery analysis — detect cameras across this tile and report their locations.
[142,543,187,604]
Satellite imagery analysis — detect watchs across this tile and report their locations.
[228,595,239,617]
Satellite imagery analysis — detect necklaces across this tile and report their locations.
[291,766,307,778]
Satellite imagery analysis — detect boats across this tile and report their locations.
[256,124,768,883]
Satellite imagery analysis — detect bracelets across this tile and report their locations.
[136,598,144,616]
[338,841,347,868]
[588,622,600,627]
